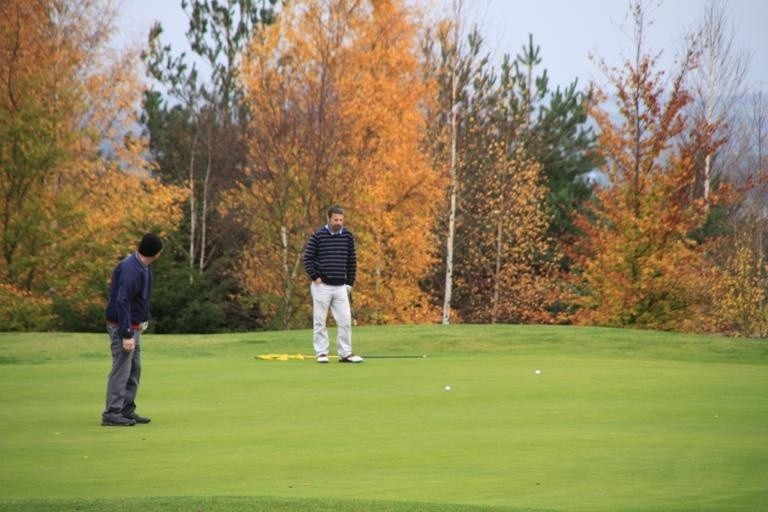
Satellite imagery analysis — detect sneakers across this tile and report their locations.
[317,354,329,362]
[102,415,136,426]
[123,411,151,423]
[339,353,363,363]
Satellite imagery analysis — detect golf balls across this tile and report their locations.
[534,368,540,373]
[444,386,449,389]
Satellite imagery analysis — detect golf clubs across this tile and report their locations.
[349,293,366,357]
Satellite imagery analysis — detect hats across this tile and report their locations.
[139,233,162,257]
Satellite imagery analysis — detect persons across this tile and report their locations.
[99,233,164,428]
[300,206,366,365]
